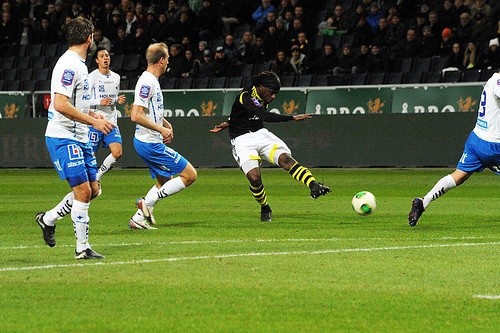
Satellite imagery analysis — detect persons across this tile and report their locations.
[210,71,331,221]
[87,47,123,197]
[35,18,117,259]
[409,71,500,226]
[128,42,198,231]
[0,0,500,79]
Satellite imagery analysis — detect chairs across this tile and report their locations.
[0,0,500,92]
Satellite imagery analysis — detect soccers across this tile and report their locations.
[351,190,378,216]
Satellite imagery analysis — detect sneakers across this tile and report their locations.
[261,207,272,222]
[74,248,105,260]
[310,182,330,199]
[35,212,56,247]
[128,218,156,229]
[136,197,156,225]
[409,197,425,226]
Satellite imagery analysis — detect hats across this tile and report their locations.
[442,28,452,37]
[112,10,120,14]
[215,47,224,52]
[489,39,499,48]
[342,44,350,49]
[203,50,211,56]
[291,45,299,52]
